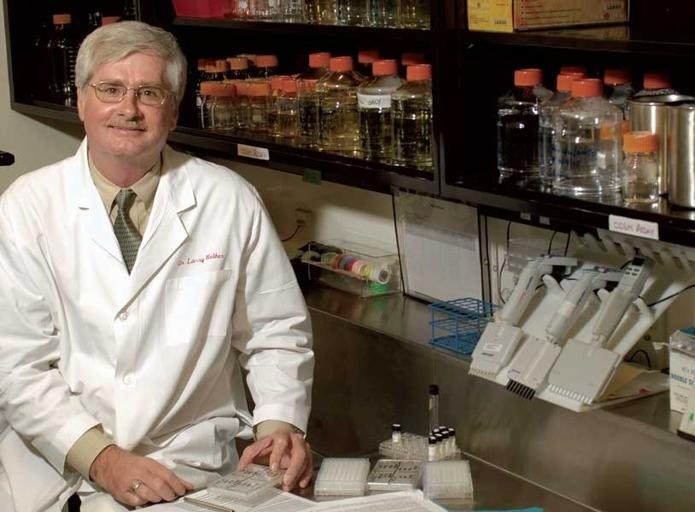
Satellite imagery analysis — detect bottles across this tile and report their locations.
[196,45,432,168]
[44,8,125,107]
[428,425,458,461]
[428,384,439,433]
[497,61,694,204]
[391,422,402,443]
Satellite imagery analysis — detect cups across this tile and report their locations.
[230,0,425,29]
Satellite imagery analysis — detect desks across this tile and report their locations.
[130,435,601,512]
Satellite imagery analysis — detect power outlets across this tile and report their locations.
[294,207,315,230]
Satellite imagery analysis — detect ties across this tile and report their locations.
[113,188,144,276]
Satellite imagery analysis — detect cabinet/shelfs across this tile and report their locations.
[136,1,442,201]
[439,2,695,251]
[428,298,505,356]
[0,0,137,130]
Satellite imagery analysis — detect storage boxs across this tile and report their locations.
[463,1,631,37]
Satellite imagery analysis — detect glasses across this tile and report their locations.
[85,81,178,107]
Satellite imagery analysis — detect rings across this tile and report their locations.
[131,479,142,491]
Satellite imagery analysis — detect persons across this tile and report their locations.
[0,20,315,512]
